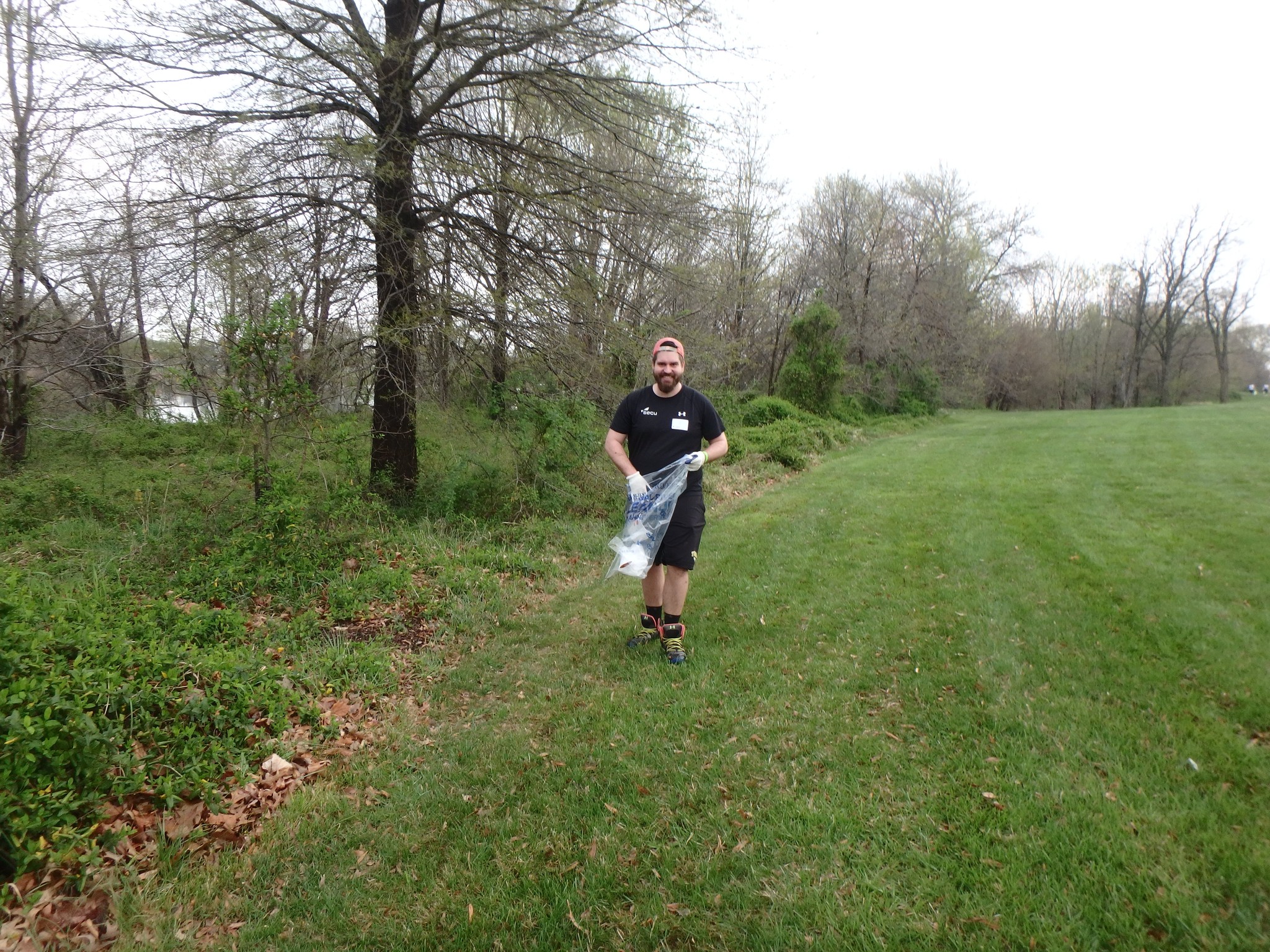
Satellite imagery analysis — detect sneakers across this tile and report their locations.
[626,613,664,650]
[659,622,686,668]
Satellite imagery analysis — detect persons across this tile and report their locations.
[604,336,730,663]
[1248,384,1269,395]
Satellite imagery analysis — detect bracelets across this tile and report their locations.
[703,451,708,464]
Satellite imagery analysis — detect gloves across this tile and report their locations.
[625,471,653,505]
[684,451,705,471]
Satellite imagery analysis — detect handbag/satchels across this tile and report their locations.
[603,454,694,581]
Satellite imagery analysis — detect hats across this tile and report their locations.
[653,337,684,360]
